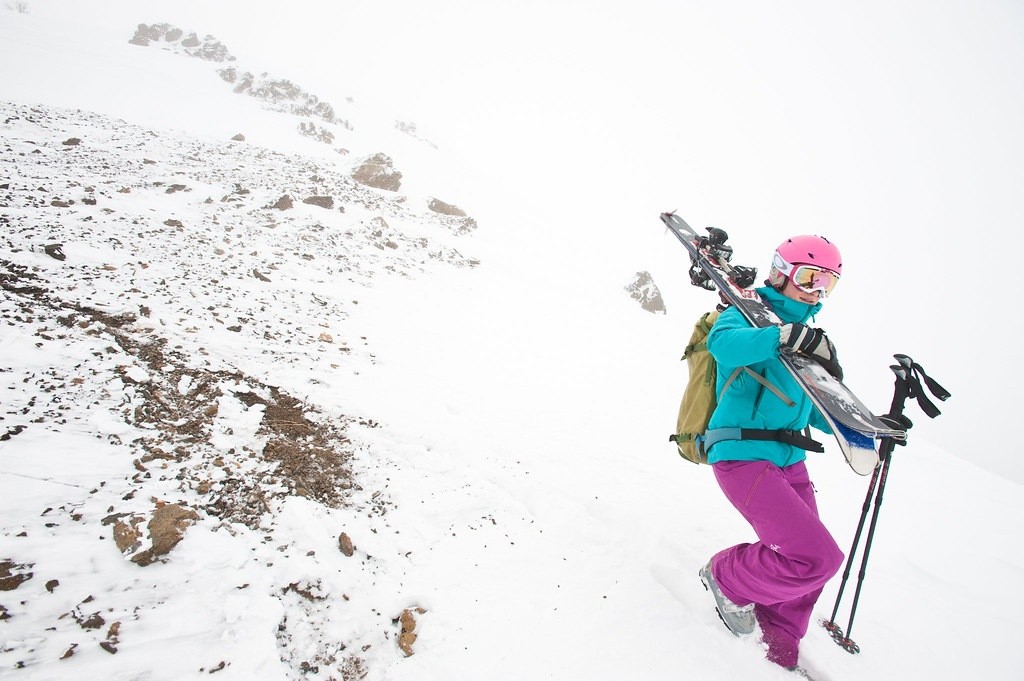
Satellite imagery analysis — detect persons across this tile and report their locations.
[698,235,912,681]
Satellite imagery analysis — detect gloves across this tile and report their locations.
[876,414,912,447]
[786,322,844,382]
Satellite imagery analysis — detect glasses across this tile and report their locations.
[790,265,838,299]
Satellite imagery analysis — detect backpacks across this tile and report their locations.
[668,310,825,465]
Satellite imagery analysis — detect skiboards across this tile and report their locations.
[661,212,907,477]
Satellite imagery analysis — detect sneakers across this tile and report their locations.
[790,666,814,681]
[700,557,755,637]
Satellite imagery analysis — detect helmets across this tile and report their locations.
[769,235,843,291]
